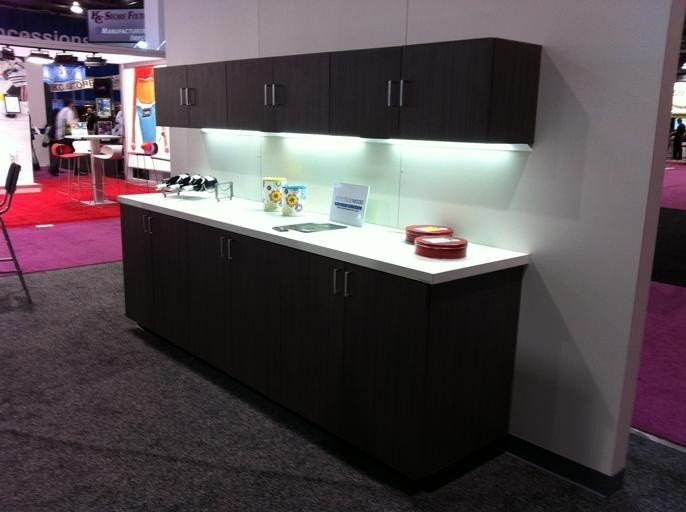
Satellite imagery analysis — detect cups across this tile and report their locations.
[261,176,308,219]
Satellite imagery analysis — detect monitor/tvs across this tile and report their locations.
[97,120,112,135]
[95,97,114,119]
[4,96,21,118]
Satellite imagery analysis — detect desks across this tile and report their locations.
[64,135,124,206]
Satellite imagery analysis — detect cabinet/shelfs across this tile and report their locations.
[118,204,522,496]
[156,40,539,147]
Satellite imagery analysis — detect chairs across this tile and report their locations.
[0,163,35,307]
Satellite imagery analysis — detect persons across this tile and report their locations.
[48,96,88,176]
[86,107,100,131]
[113,105,122,132]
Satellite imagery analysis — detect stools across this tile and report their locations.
[52,143,158,207]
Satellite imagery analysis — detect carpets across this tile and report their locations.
[0,261,686,510]
[0,175,171,229]
[0,165,166,273]
[631,161,686,447]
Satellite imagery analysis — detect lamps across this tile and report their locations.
[0,44,107,68]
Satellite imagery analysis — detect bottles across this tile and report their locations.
[134,64,157,153]
